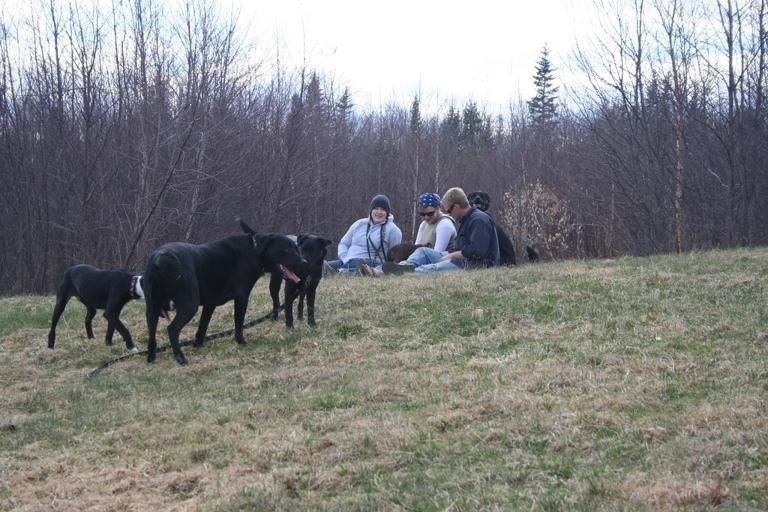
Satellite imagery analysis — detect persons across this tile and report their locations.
[323,186,501,277]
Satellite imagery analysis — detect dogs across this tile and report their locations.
[46,262,177,356]
[386,241,435,264]
[270,230,333,333]
[143,230,309,370]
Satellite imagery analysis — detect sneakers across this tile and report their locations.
[323,260,338,275]
[382,261,415,275]
[361,264,374,276]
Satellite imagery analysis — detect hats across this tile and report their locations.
[370,194,390,215]
[418,193,441,208]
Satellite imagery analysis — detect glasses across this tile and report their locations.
[421,212,434,217]
[446,203,455,215]
